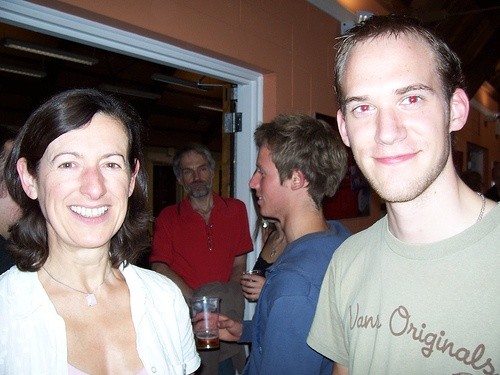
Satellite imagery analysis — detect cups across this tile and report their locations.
[241,269,262,302]
[189,296,222,349]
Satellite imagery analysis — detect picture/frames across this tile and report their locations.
[314,111,371,222]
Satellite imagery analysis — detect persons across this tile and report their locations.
[0,89,204,375]
[460,160,500,203]
[191,112,349,375]
[325,18,500,375]
[149,144,252,375]
[0,134,26,274]
[239,170,288,303]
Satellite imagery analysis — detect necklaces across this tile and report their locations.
[476,192,486,221]
[270,233,285,256]
[37,263,112,307]
[193,194,212,214]
